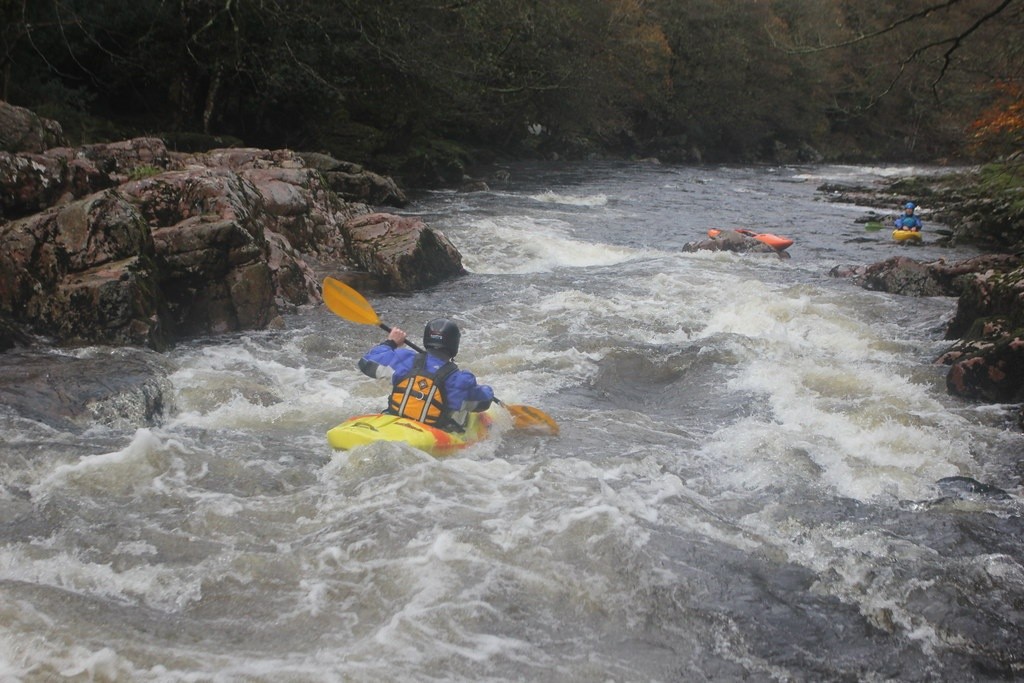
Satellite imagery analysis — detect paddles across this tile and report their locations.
[863,221,954,236]
[320,276,561,435]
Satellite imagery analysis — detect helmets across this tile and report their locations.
[906,202,915,209]
[424,318,461,357]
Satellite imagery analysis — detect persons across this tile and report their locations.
[358,318,494,434]
[894,203,922,231]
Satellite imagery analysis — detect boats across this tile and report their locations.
[893,228,922,241]
[326,410,496,458]
[708,228,794,252]
